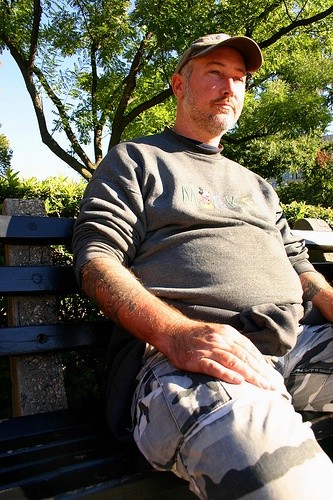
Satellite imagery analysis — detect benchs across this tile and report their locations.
[0,199,333,500]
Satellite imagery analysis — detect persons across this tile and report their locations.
[69,33,333,500]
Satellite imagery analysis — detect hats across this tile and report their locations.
[175,33,263,73]
